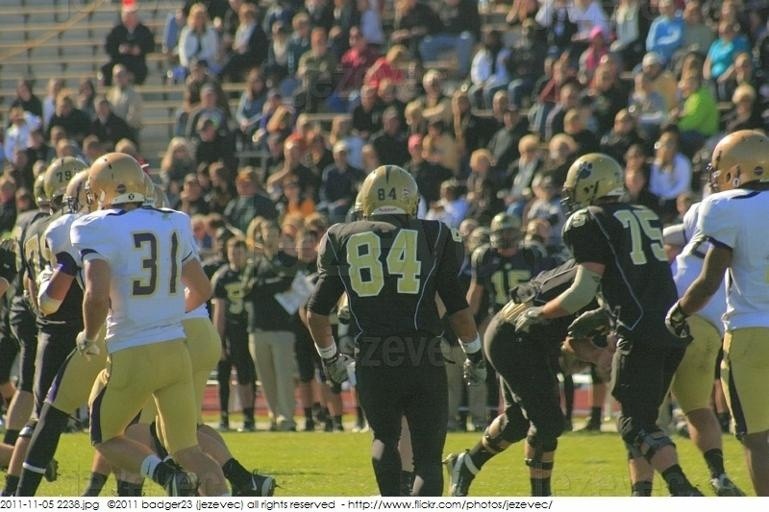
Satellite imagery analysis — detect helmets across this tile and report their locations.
[43,156,89,202]
[560,153,624,205]
[145,170,169,208]
[61,170,89,207]
[33,172,50,206]
[489,212,521,249]
[355,165,419,216]
[708,129,769,190]
[88,152,145,204]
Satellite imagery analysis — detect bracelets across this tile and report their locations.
[313,335,337,359]
[455,331,483,355]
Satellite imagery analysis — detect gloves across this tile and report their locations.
[458,360,488,386]
[515,307,543,332]
[665,302,689,338]
[76,331,100,360]
[313,352,353,393]
[568,310,615,343]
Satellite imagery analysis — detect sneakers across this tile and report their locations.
[45,460,58,481]
[170,472,200,496]
[442,453,474,497]
[712,475,744,496]
[244,474,275,496]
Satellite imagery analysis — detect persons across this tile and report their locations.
[97,1,155,86]
[304,164,484,496]
[464,211,550,328]
[620,0,769,149]
[4,170,52,448]
[662,130,768,497]
[405,132,582,224]
[14,167,175,496]
[1,243,17,425]
[366,0,620,153]
[1,155,113,496]
[189,212,367,434]
[442,255,614,496]
[441,217,492,431]
[574,335,614,433]
[656,132,751,496]
[1,441,61,481]
[514,152,705,497]
[1,77,37,240]
[162,1,367,213]
[621,132,700,224]
[69,151,229,496]
[156,168,278,497]
[37,63,148,169]
[522,216,575,433]
